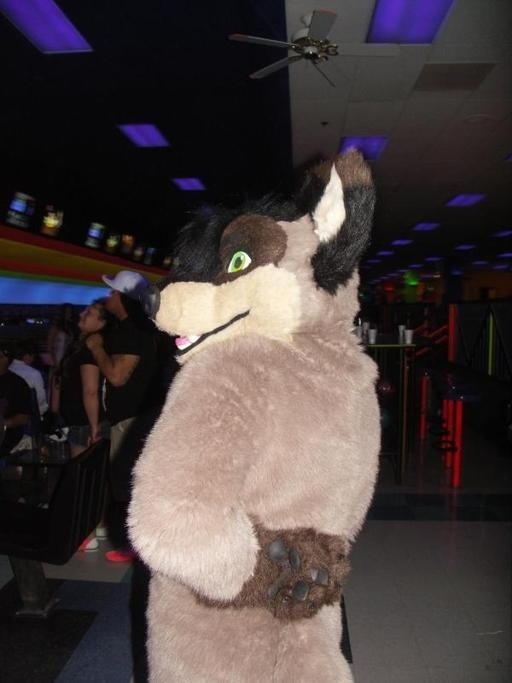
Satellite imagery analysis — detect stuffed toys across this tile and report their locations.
[124,142,383,683]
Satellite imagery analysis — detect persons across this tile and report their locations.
[0,268,181,622]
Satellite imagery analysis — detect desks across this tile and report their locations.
[365,335,416,487]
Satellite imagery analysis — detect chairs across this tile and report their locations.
[1,440,111,620]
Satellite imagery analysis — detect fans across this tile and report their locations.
[227,8,398,88]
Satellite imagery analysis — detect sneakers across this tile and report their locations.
[85,527,133,562]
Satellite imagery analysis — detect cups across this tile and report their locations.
[398,324,405,335]
[367,328,377,344]
[403,329,414,344]
[362,321,370,334]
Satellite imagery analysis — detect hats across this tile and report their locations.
[101,271,146,301]
